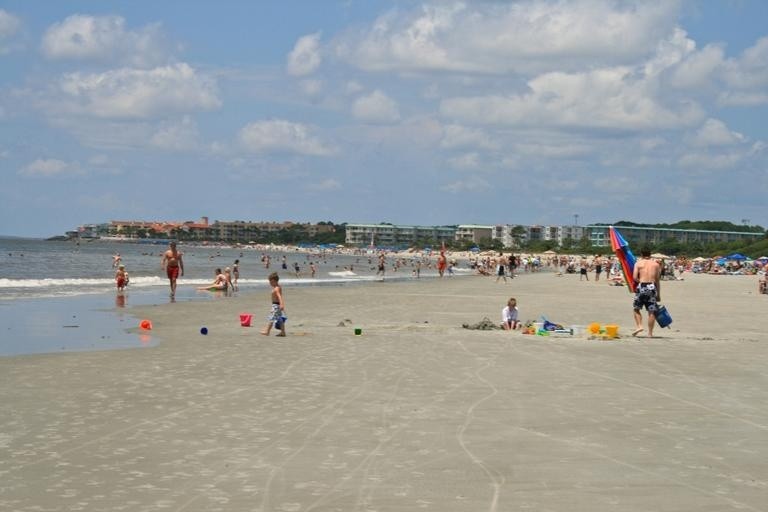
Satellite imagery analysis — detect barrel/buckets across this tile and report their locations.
[239,314,252,326]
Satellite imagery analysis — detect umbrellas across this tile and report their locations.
[608,225,673,330]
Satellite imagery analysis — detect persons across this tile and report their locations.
[140,242,184,301]
[110,252,130,291]
[260,273,288,338]
[631,245,662,339]
[502,298,521,330]
[195,240,767,299]
